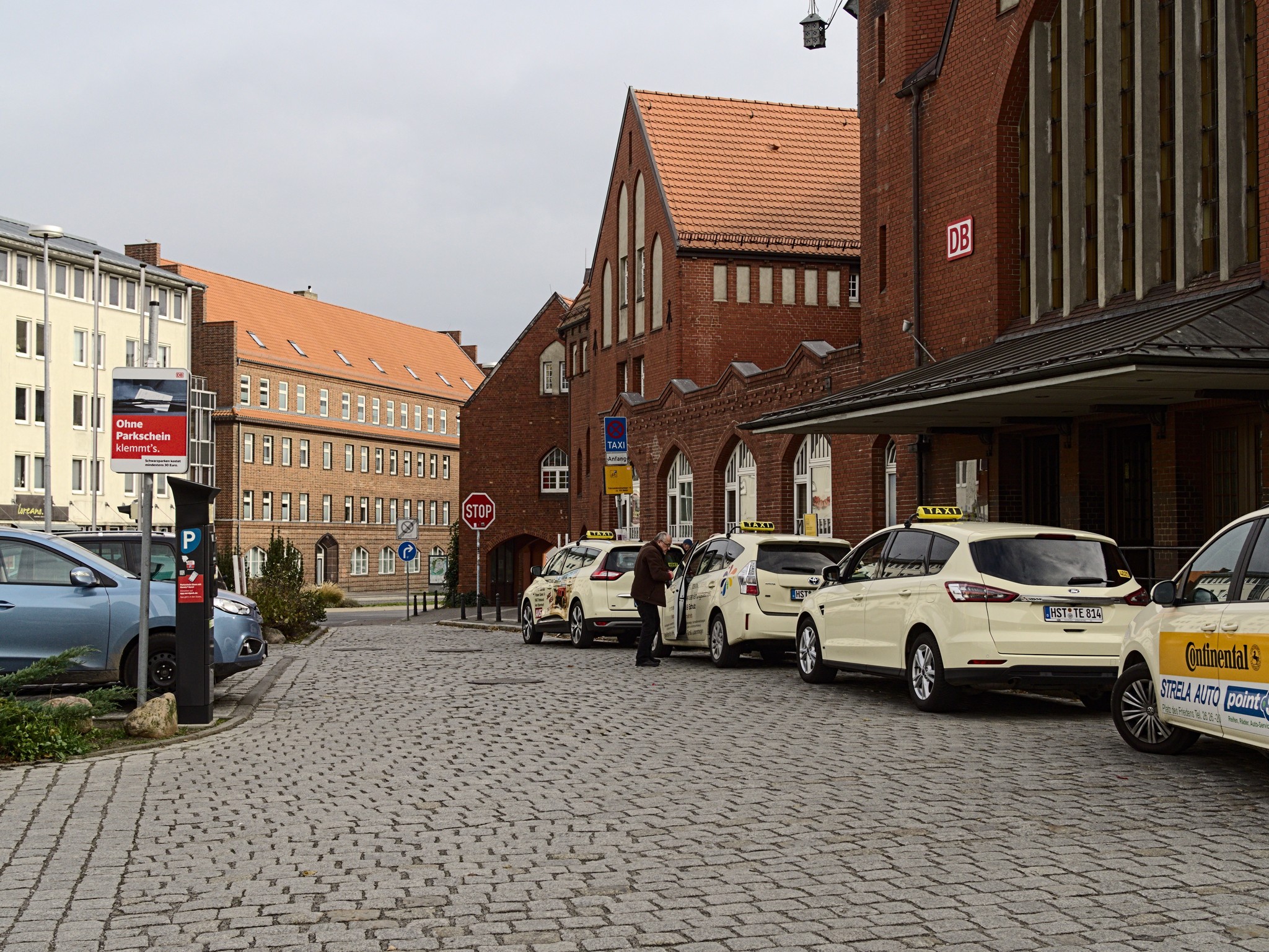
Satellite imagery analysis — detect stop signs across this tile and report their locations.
[462,492,496,530]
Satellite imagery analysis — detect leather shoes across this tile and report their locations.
[635,657,661,667]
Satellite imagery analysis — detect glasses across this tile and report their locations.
[660,540,671,547]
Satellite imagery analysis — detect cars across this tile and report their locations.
[1,525,266,701]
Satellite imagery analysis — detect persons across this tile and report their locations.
[630,530,675,667]
[674,539,693,574]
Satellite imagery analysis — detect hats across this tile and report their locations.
[683,539,693,547]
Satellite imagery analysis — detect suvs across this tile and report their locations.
[520,529,687,649]
[650,520,872,668]
[1111,507,1269,755]
[793,505,1152,711]
[0,531,228,593]
